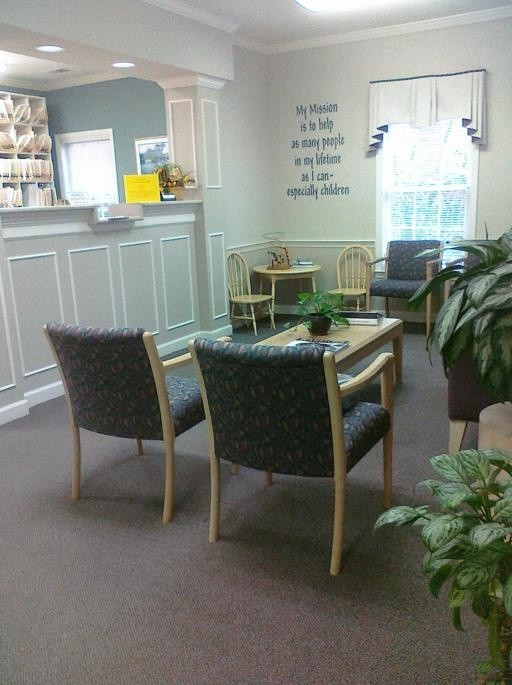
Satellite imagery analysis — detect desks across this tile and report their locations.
[253,262,321,329]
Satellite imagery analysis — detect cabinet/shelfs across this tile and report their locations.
[0,92,56,208]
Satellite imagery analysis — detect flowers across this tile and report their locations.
[152,163,192,187]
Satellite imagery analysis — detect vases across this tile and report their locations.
[168,187,184,201]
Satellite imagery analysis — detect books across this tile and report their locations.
[284,338,350,356]
[334,311,384,325]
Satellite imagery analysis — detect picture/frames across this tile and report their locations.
[134,135,169,175]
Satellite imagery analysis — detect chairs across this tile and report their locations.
[327,244,374,312]
[226,252,276,337]
[185,337,393,577]
[446,239,512,462]
[43,322,207,524]
[365,240,451,338]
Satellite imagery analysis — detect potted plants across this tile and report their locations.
[281,290,350,336]
